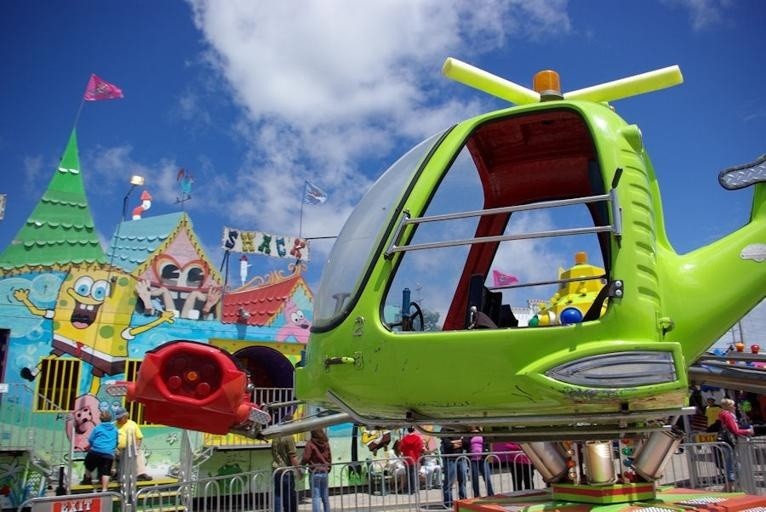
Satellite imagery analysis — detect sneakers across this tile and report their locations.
[79,474,92,486]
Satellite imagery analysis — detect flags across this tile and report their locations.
[301,182,329,208]
[83,77,125,102]
[494,270,520,286]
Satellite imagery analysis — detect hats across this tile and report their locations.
[114,407,128,419]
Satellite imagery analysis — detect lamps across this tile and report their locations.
[122,175,145,220]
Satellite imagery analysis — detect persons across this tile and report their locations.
[464,422,496,498]
[79,411,118,493]
[505,441,536,491]
[720,398,756,482]
[270,414,306,511]
[396,423,425,493]
[389,439,409,493]
[706,396,726,469]
[439,417,468,506]
[300,426,333,511]
[419,439,444,491]
[109,404,153,483]
